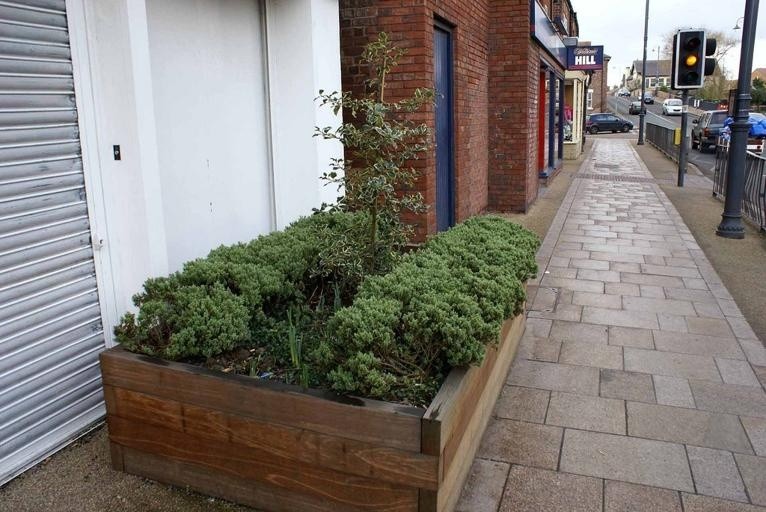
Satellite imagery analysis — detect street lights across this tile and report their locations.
[652,46,659,90]
[734,16,744,29]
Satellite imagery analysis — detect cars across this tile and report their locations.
[662,99,682,116]
[638,92,655,104]
[587,112,633,134]
[618,90,631,97]
[628,102,646,114]
[691,110,729,153]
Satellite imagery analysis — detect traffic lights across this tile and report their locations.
[675,28,717,88]
